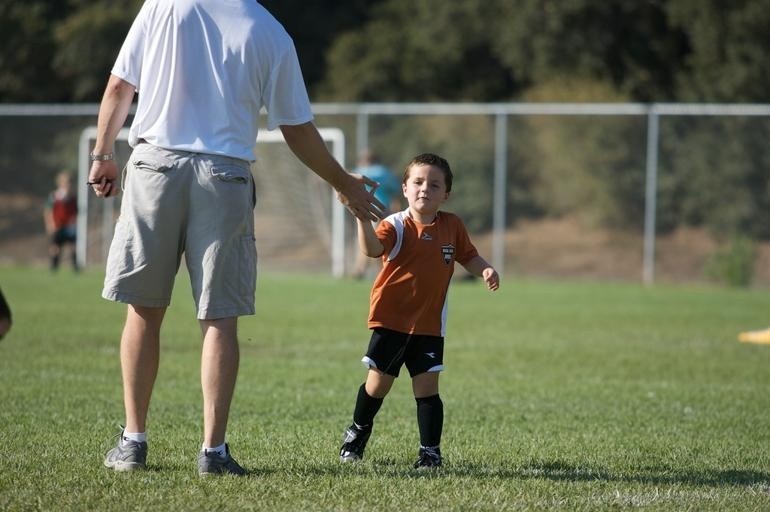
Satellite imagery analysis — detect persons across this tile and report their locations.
[0,286,12,340]
[337,152,503,473]
[42,169,83,274]
[88,1,387,477]
[345,152,402,281]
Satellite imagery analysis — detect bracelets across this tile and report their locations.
[89,148,117,161]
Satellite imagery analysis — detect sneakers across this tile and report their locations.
[414,444,442,469]
[197,443,244,476]
[338,423,373,465]
[104,423,148,474]
